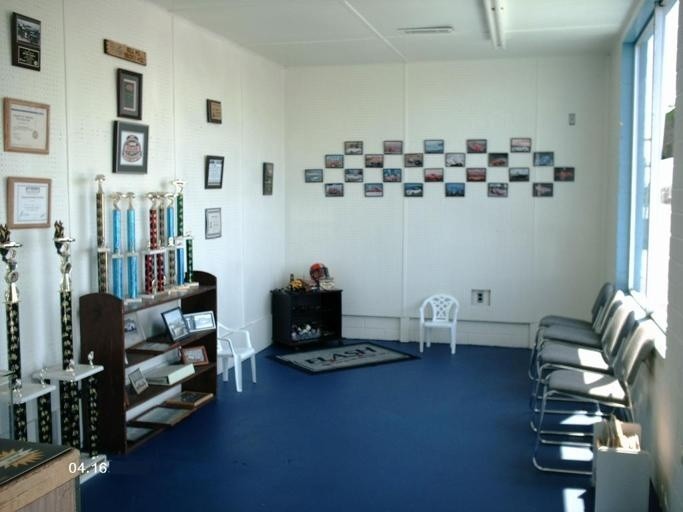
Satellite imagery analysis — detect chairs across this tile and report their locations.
[529,283,623,397]
[420,294,459,355]
[523,301,641,436]
[534,292,641,401]
[531,321,666,474]
[217,321,257,392]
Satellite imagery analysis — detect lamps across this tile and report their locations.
[396,28,453,33]
[482,0,506,49]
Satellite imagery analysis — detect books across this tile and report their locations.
[146,363,195,386]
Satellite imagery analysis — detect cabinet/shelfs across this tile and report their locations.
[79,272,216,453]
[270,286,343,349]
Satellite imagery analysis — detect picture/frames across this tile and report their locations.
[554,167,575,181]
[535,152,554,166]
[511,138,530,152]
[6,176,52,229]
[129,405,196,427]
[364,183,383,197]
[365,155,384,167]
[263,162,274,196]
[208,98,221,122]
[182,346,211,366]
[116,121,149,173]
[404,183,423,196]
[325,183,344,197]
[345,169,363,182]
[383,168,401,181]
[13,13,42,70]
[125,313,146,348]
[117,68,143,119]
[489,153,509,167]
[4,96,50,155]
[383,141,402,154]
[404,153,423,167]
[184,311,216,333]
[467,140,487,152]
[446,182,465,197]
[467,168,486,182]
[160,307,193,341]
[445,153,465,167]
[325,154,344,168]
[205,208,222,239]
[423,140,444,153]
[344,141,363,155]
[205,155,224,189]
[128,368,149,394]
[509,167,529,182]
[425,169,443,182]
[167,390,213,406]
[304,169,323,183]
[489,183,508,197]
[126,427,153,442]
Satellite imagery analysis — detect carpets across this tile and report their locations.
[266,341,421,376]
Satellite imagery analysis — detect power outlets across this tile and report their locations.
[472,289,491,307]
